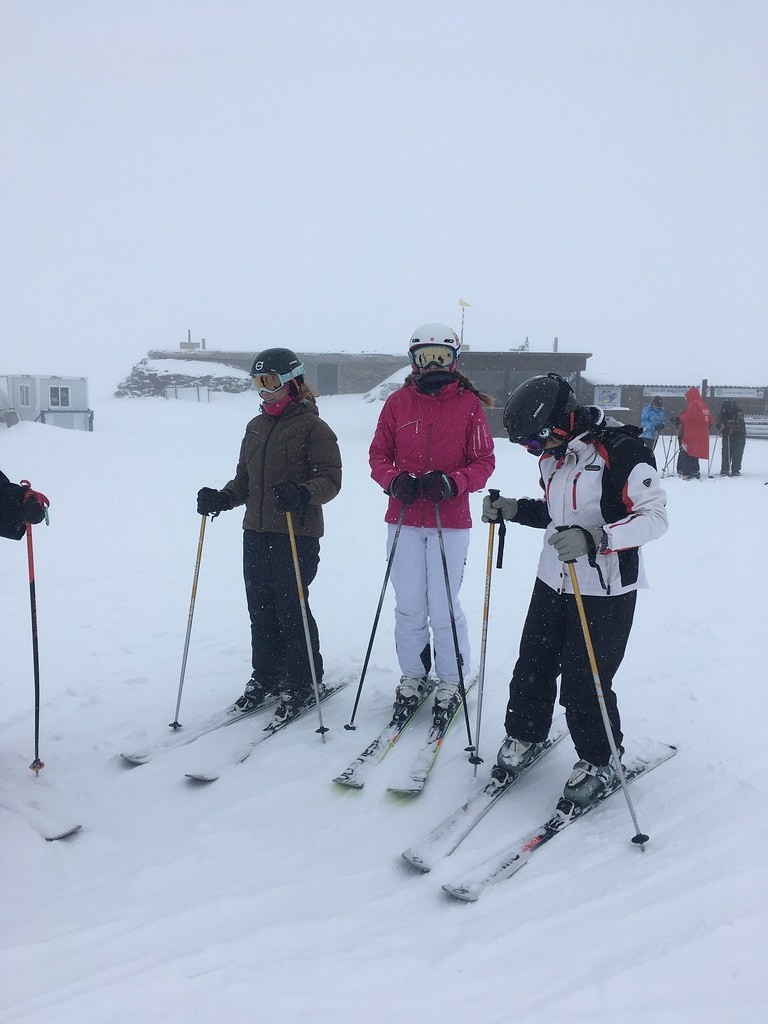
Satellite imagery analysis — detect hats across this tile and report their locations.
[652,396,663,406]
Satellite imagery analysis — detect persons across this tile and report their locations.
[482,374,669,812]
[0,471,45,541]
[638,396,668,451]
[369,324,494,720]
[716,398,746,475]
[197,348,342,723]
[670,387,712,480]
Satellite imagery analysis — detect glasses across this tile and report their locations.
[251,373,284,393]
[412,345,457,369]
[521,438,546,457]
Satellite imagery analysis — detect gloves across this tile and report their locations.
[548,526,602,562]
[23,491,45,524]
[482,495,517,524]
[422,470,457,500]
[392,472,418,504]
[671,416,682,424]
[655,422,665,431]
[269,481,309,512]
[196,488,230,516]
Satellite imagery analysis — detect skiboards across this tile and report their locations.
[334,657,480,795]
[0,784,82,844]
[403,710,679,903]
[118,655,365,783]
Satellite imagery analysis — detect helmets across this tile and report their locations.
[503,374,574,444]
[408,323,460,373]
[250,348,303,393]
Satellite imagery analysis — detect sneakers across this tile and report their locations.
[434,682,462,710]
[232,673,289,712]
[275,681,325,718]
[497,733,542,776]
[397,672,428,705]
[563,747,625,806]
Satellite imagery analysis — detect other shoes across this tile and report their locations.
[721,470,730,476]
[732,470,740,476]
